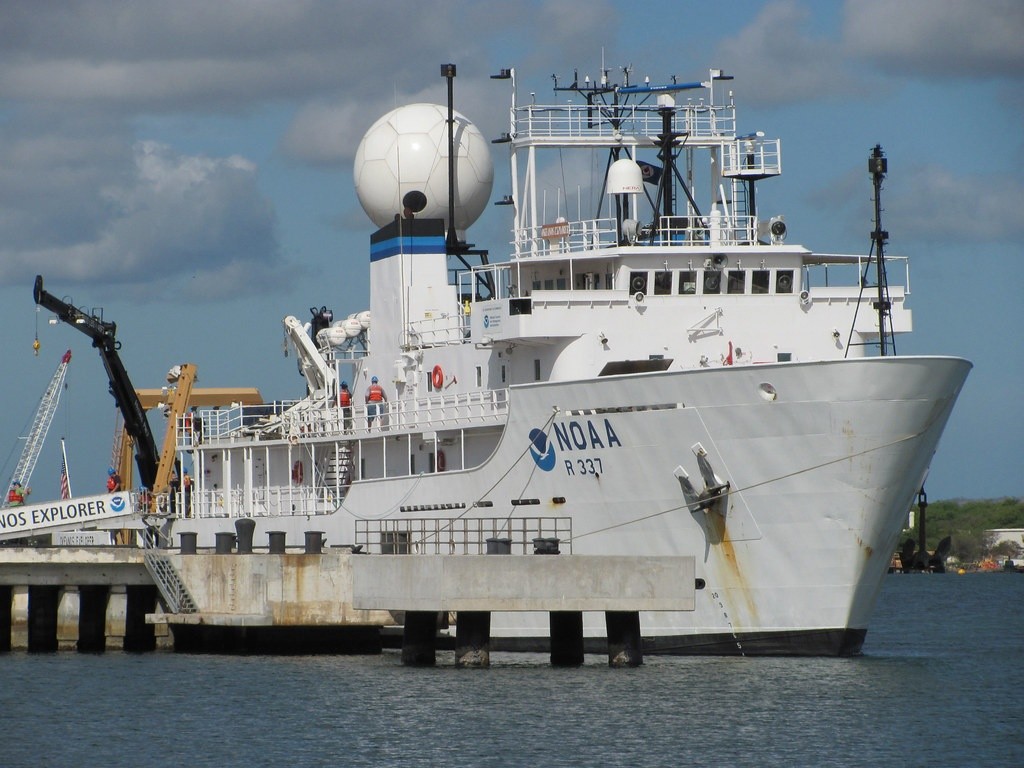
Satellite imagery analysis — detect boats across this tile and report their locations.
[136,61,975,653]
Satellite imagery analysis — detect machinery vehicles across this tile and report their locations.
[30,272,262,542]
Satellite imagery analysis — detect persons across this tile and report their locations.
[183,466,192,517]
[106,466,122,494]
[333,381,353,435]
[7,479,31,508]
[365,375,388,432]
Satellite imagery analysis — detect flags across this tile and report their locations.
[61,441,70,500]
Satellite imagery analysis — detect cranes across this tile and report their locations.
[1,347,72,513]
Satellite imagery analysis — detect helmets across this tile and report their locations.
[339,382,348,387]
[371,376,378,382]
[183,466,189,473]
[107,468,115,475]
[14,481,20,485]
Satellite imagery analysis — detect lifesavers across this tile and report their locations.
[187,412,193,433]
[430,364,445,390]
[292,460,303,486]
[432,451,445,473]
